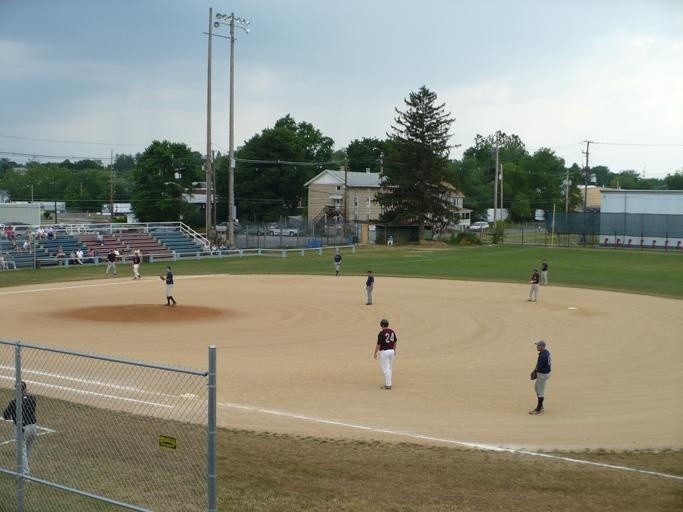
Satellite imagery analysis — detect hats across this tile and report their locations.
[534,341,545,346]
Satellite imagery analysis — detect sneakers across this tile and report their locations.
[529,409,540,414]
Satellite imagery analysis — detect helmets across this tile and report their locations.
[380,320,388,327]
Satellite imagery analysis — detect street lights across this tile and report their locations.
[26,183,34,202]
[212,11,255,251]
[164,181,181,187]
[370,144,386,215]
[190,181,206,186]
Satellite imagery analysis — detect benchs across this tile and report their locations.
[0,226,204,268]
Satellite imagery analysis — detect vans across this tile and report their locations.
[469,221,489,232]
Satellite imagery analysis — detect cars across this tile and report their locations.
[212,219,243,234]
[244,222,298,236]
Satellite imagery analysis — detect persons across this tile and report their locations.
[0,224,141,281]
[3,381,37,480]
[529,341,552,414]
[365,271,374,305]
[540,259,548,286]
[526,268,539,302]
[160,266,176,307]
[334,249,343,276]
[203,240,231,256]
[374,319,397,390]
